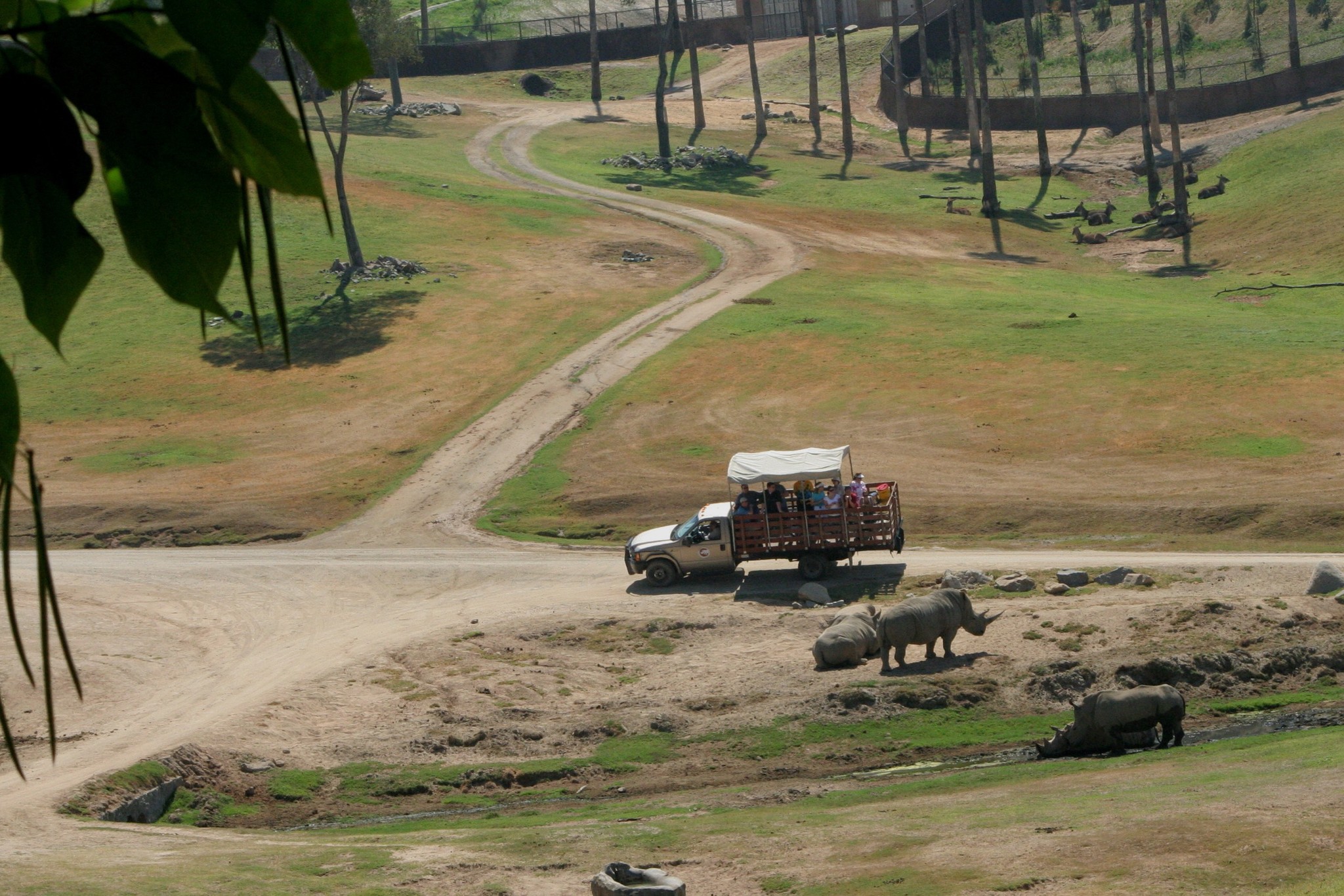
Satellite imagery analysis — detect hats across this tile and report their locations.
[816,482,827,488]
[845,486,851,490]
[740,497,748,502]
[855,474,864,478]
[831,476,841,484]
[774,478,782,482]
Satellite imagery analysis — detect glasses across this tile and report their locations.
[829,489,834,492]
[711,525,714,527]
[742,486,749,489]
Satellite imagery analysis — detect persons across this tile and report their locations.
[704,520,721,541]
[735,477,879,547]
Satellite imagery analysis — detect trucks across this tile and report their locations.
[624,478,906,588]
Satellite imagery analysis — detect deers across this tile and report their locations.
[946,197,972,216]
[1072,225,1107,244]
[1198,174,1231,199]
[1183,161,1198,185]
[1131,188,1196,239]
[1074,200,1117,226]
